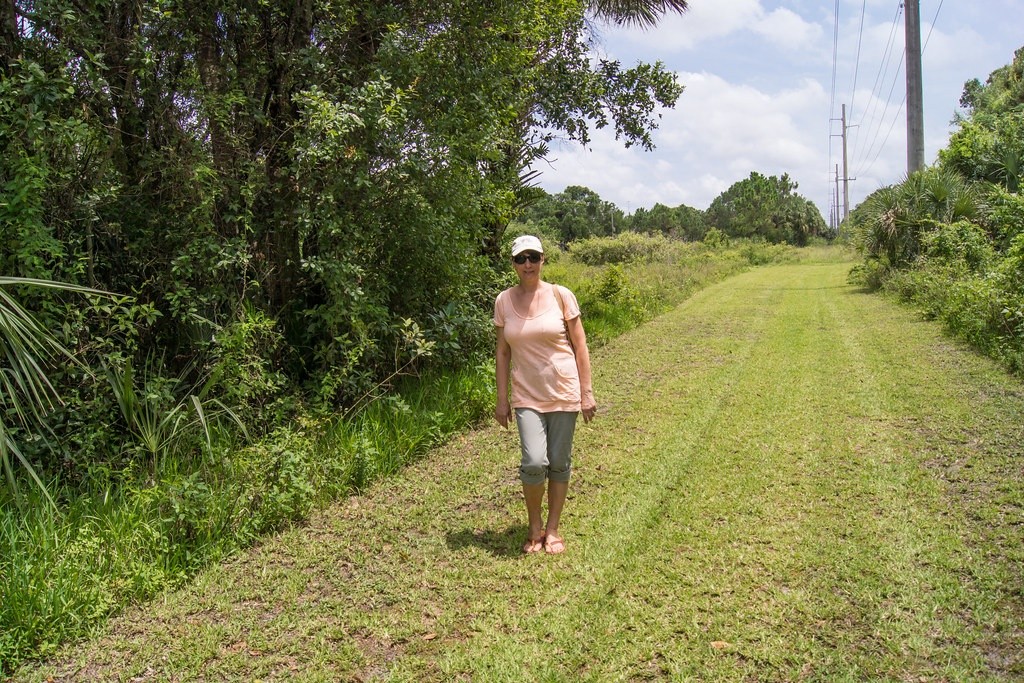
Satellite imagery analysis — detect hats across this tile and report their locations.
[511,234,543,257]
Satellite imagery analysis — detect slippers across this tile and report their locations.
[544,532,565,555]
[523,537,544,552]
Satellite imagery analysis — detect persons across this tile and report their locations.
[492,235,597,552]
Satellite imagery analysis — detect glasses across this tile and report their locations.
[514,254,542,264]
